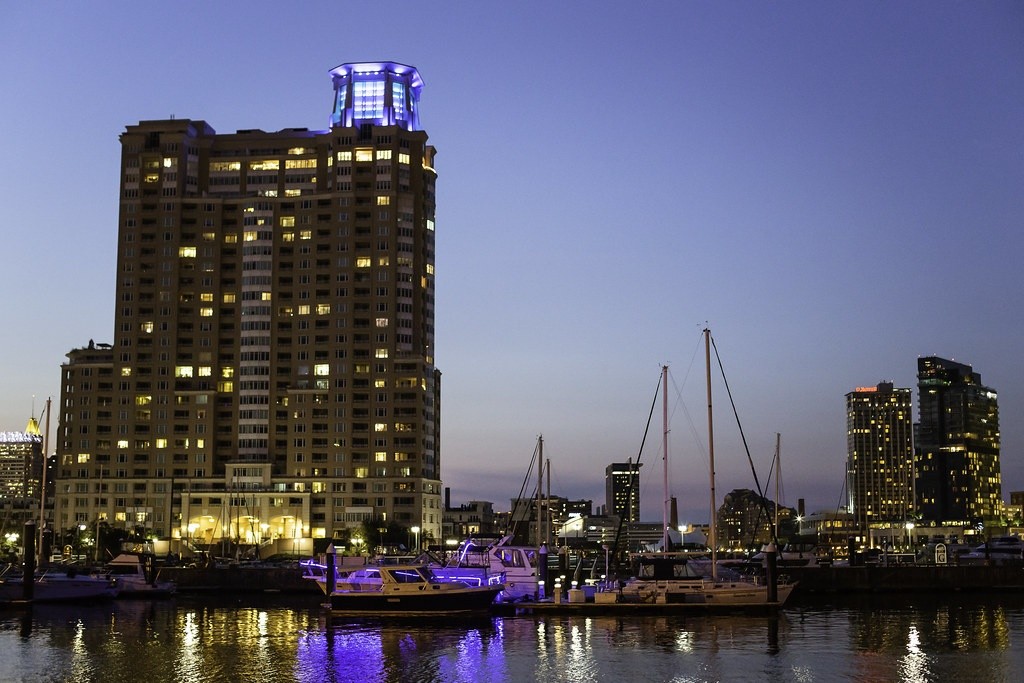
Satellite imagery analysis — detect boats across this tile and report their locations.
[959,536,1024,565]
[429,540,540,602]
[313,556,505,618]
[104,549,177,592]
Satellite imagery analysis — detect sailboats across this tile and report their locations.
[600,323,800,611]
[1,397,116,600]
[747,429,825,565]
[508,433,600,580]
[163,469,282,593]
[272,489,331,595]
